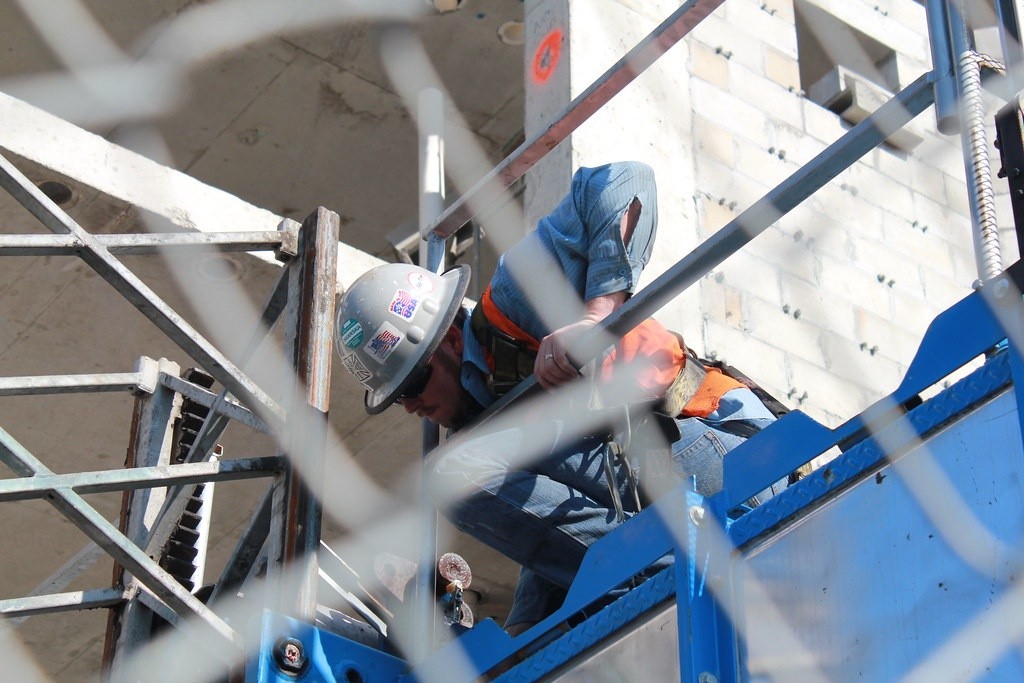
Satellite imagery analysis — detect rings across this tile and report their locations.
[543,354,553,360]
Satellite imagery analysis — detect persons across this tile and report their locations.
[335,160,798,636]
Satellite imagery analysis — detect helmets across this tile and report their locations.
[334,262,472,414]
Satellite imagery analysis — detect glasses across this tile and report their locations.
[393,352,436,406]
